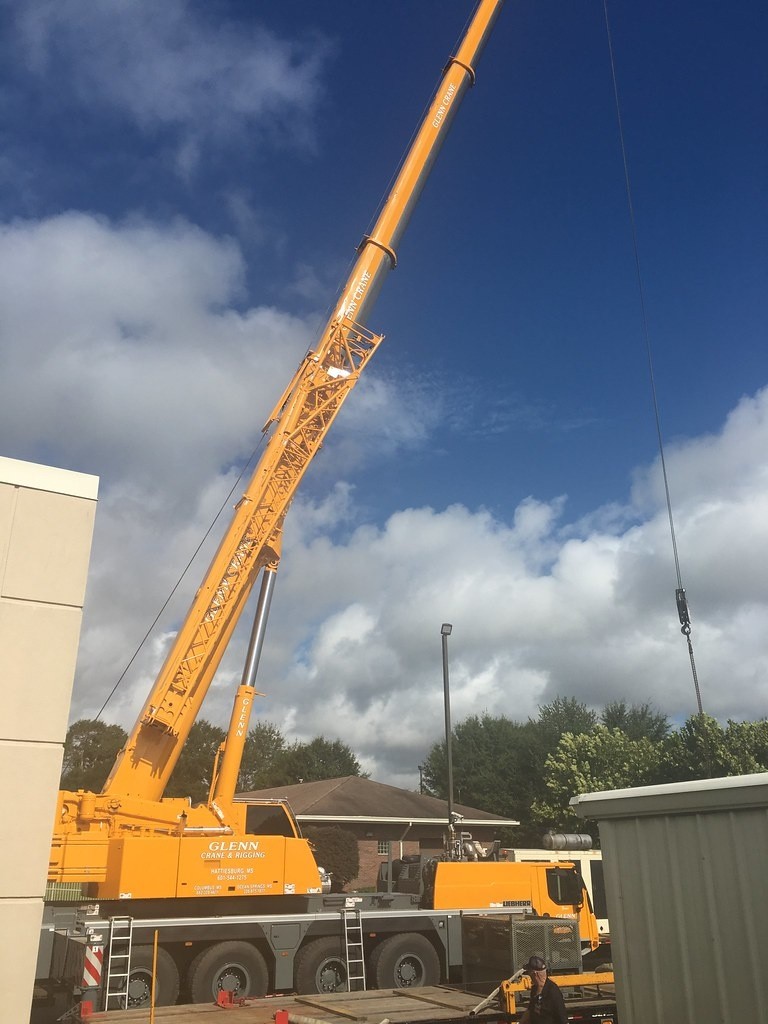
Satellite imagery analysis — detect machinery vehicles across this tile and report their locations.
[34,0,605,1021]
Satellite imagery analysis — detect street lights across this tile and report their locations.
[418,766,426,795]
[439,624,453,830]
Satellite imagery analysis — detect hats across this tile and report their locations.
[523,956,546,971]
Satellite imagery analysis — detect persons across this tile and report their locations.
[518,956,569,1024]
